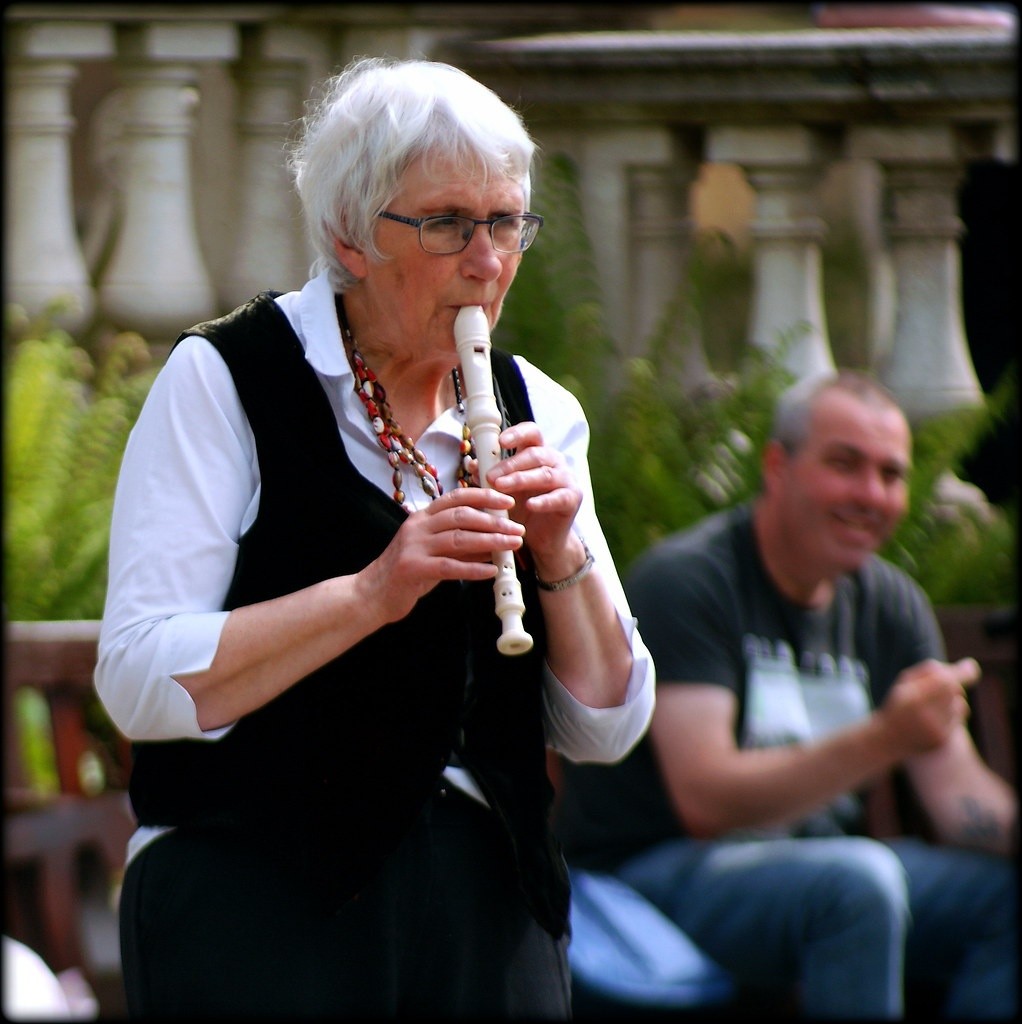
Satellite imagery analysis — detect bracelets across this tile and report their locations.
[534,536,596,591]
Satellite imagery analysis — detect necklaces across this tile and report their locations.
[333,289,481,509]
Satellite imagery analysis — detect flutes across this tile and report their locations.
[452,304,532,654]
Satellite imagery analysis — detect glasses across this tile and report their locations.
[371,206,545,257]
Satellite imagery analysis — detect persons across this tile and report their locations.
[572,371,1022,1024]
[92,58,658,1024]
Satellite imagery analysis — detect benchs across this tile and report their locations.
[4,608,1017,1015]
